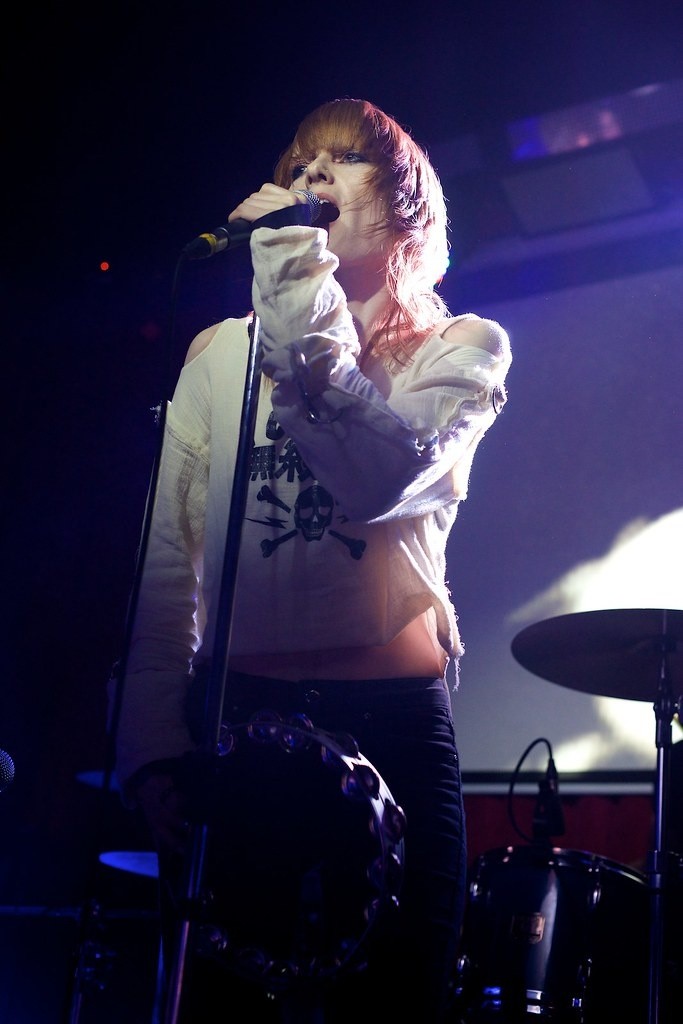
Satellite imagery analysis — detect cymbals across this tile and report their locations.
[167,705,413,983]
[509,608,682,701]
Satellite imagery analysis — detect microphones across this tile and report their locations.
[540,758,565,838]
[184,189,321,260]
[1,748,14,794]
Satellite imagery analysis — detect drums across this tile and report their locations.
[453,845,654,1023]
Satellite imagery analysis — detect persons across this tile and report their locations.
[118,99,513,1024]
